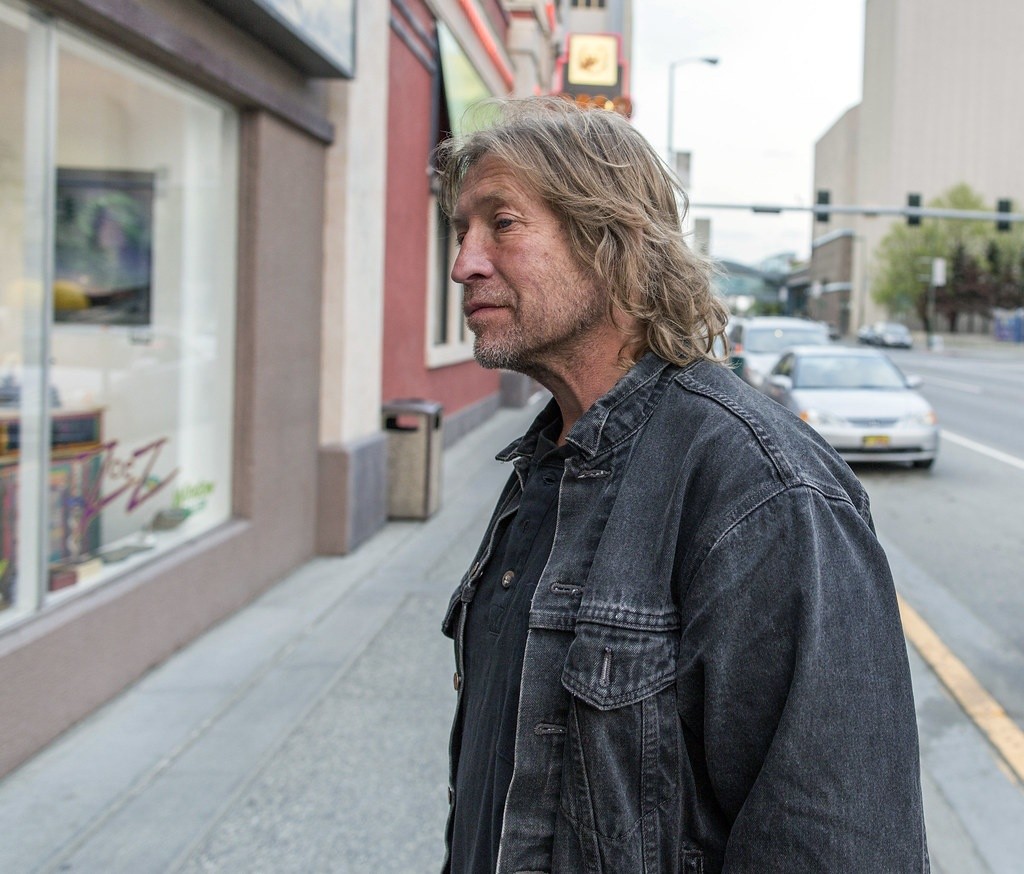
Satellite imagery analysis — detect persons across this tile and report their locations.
[438,95,930,874]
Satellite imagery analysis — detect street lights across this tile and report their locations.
[666,54,720,170]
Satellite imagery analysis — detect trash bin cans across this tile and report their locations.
[381,397,444,524]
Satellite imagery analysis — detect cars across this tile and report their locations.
[722,316,833,391]
[760,345,940,470]
[856,318,915,350]
